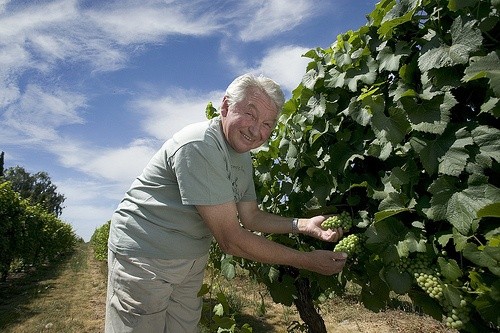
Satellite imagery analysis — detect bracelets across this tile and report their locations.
[292,217,298,235]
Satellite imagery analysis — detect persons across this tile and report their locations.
[104,73,348,333]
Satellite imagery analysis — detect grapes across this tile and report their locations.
[389,251,471,330]
[321,211,366,259]
